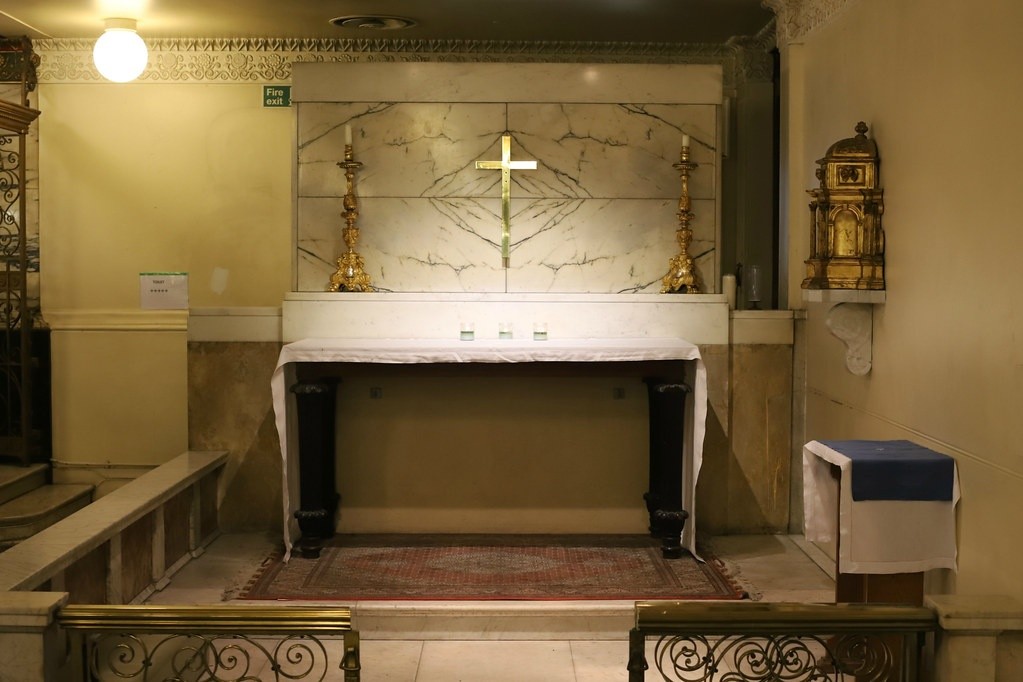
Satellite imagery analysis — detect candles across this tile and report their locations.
[500,328,512,339]
[747,267,761,300]
[721,275,737,310]
[682,132,688,147]
[344,123,352,145]
[534,328,546,340]
[460,327,473,340]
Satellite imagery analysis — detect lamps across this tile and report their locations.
[92,18,147,81]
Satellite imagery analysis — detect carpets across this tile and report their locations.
[223,542,761,601]
